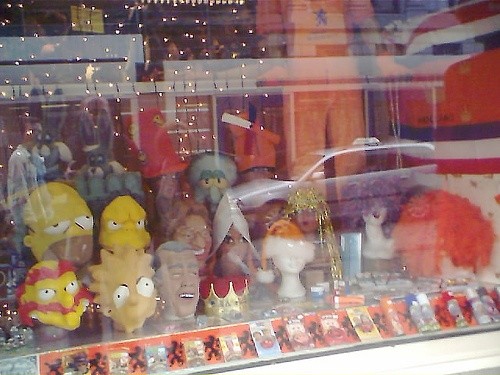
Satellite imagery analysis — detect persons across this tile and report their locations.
[63,97,139,170]
[255,0,408,211]
[8,118,47,292]
[34,10,276,82]
[153,242,201,322]
[212,194,256,275]
[283,188,343,280]
[259,218,313,299]
[161,201,214,271]
[342,179,404,260]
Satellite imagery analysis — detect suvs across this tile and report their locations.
[228,136,442,234]
